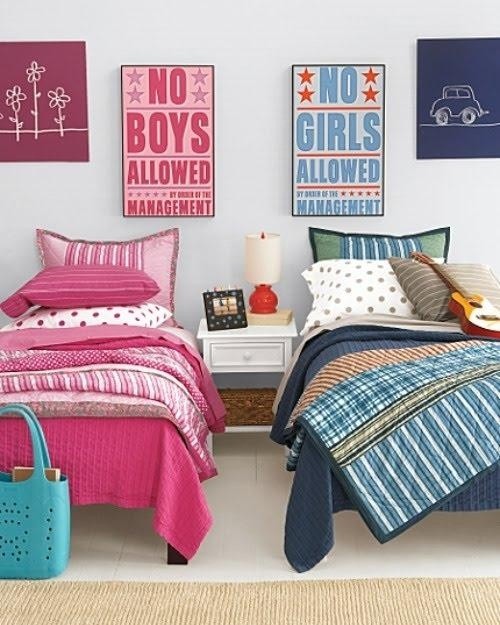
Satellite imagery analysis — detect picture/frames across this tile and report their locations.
[120,63,216,219]
[203,289,248,331]
[290,62,387,218]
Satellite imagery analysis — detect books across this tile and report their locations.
[10,466,61,483]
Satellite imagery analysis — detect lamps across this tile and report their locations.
[243,231,282,314]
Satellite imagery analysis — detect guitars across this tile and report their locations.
[410,251,500,340]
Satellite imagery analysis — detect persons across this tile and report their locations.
[225,298,231,312]
[218,298,225,313]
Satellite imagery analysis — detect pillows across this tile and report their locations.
[0,226,181,330]
[298,226,500,338]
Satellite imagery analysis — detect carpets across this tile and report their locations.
[0,579,500,625]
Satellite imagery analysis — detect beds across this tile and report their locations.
[268,320,500,572]
[0,323,227,566]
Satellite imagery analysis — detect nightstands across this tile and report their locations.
[196,319,299,459]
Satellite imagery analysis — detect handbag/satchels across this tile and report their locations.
[0,405,70,580]
